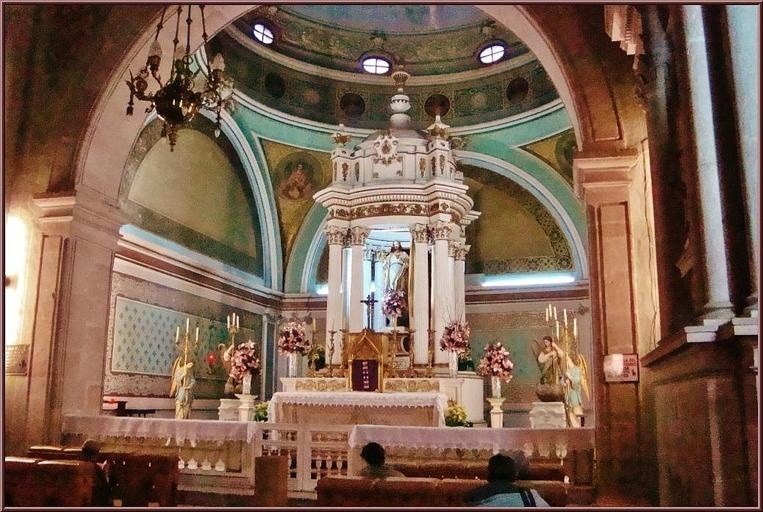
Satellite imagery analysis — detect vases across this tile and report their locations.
[448,351,458,372]
[389,313,397,328]
[287,352,298,377]
[489,376,501,398]
[242,375,251,393]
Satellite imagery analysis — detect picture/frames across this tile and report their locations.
[601,353,639,383]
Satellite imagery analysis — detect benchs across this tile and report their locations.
[315,461,566,509]
[4,446,179,508]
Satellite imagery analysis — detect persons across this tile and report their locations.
[538,335,564,384]
[559,353,585,428]
[353,442,407,478]
[223,340,248,394]
[77,438,108,493]
[463,452,551,507]
[175,358,196,419]
[383,240,410,291]
[279,162,313,199]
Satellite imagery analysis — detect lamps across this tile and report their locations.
[122,3,236,152]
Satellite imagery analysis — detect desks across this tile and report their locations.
[266,390,445,428]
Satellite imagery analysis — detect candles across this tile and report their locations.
[544,302,577,340]
[174,326,179,344]
[185,317,189,333]
[225,312,239,329]
[312,318,316,331]
[195,327,199,343]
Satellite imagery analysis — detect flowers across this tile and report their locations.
[228,339,262,377]
[276,322,310,356]
[438,320,473,361]
[475,341,513,383]
[381,288,408,317]
